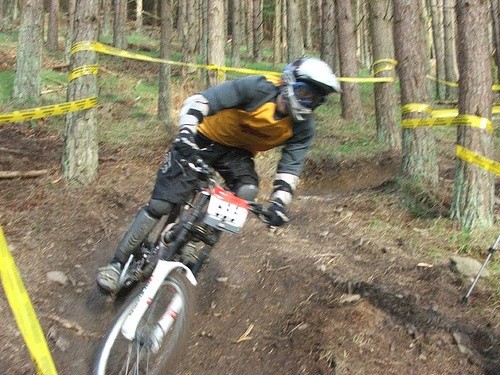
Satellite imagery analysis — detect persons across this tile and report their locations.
[95,56,343,293]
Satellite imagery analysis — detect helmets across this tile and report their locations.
[281,56,342,124]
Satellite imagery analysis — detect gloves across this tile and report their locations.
[184,154,212,183]
[262,201,286,227]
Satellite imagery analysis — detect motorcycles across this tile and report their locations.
[91,136,286,375]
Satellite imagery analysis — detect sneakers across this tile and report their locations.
[96,261,123,295]
